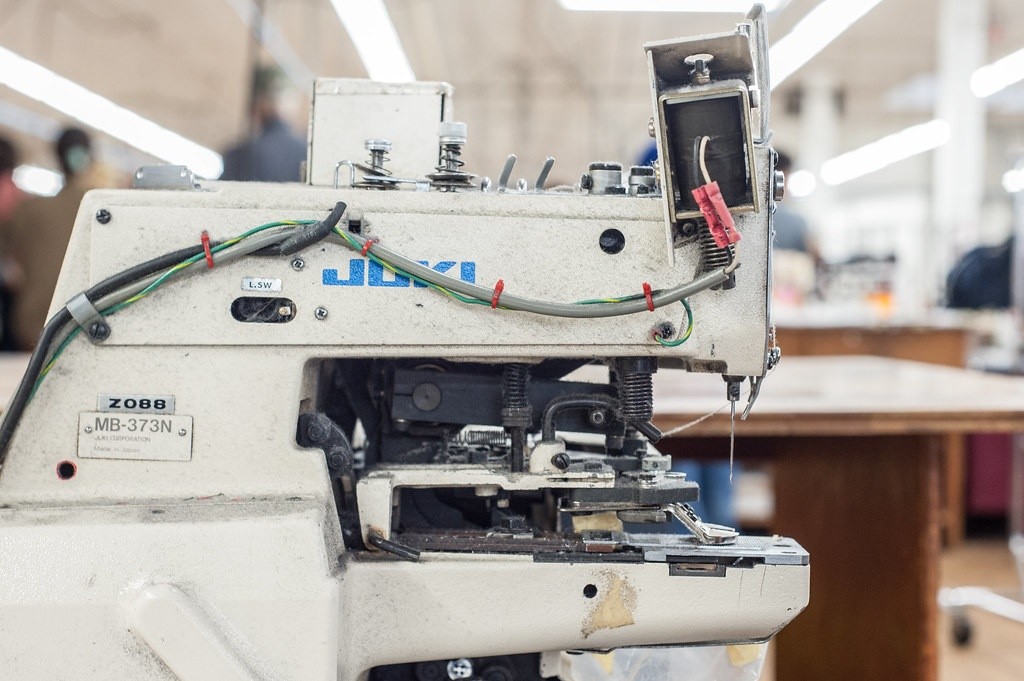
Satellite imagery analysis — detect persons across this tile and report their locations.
[0,124,97,353]
[634,131,823,534]
[217,65,308,182]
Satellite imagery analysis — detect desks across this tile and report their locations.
[559,353,1024,681]
[771,309,979,546]
